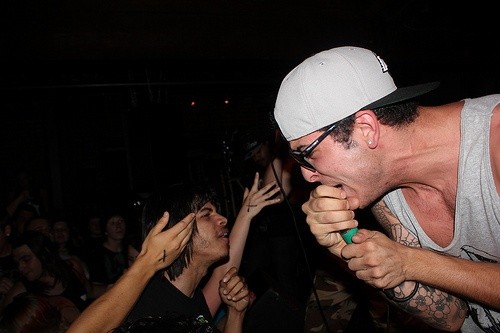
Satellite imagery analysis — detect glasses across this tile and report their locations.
[287,124,341,171]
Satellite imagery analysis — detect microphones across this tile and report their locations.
[335,226,397,299]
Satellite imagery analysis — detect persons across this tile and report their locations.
[66,181,252,333]
[274,45,500,333]
[201,171,281,318]
[1,190,145,333]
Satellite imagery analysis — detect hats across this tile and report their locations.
[273,45,437,141]
